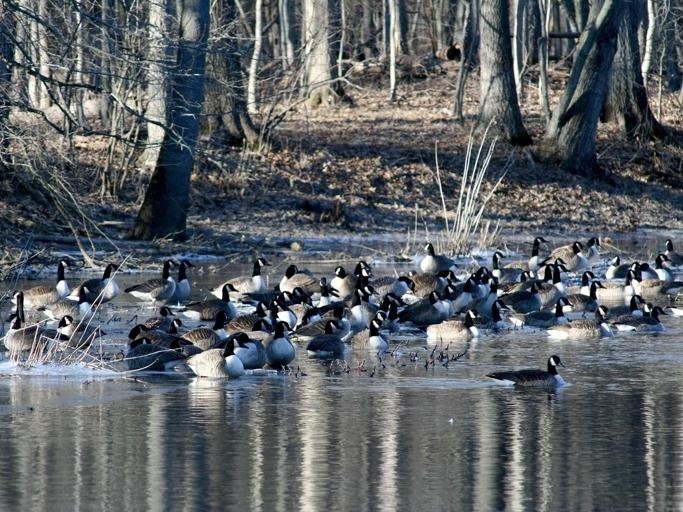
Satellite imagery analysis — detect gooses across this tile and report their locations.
[484,355,566,388]
[1,234,682,379]
